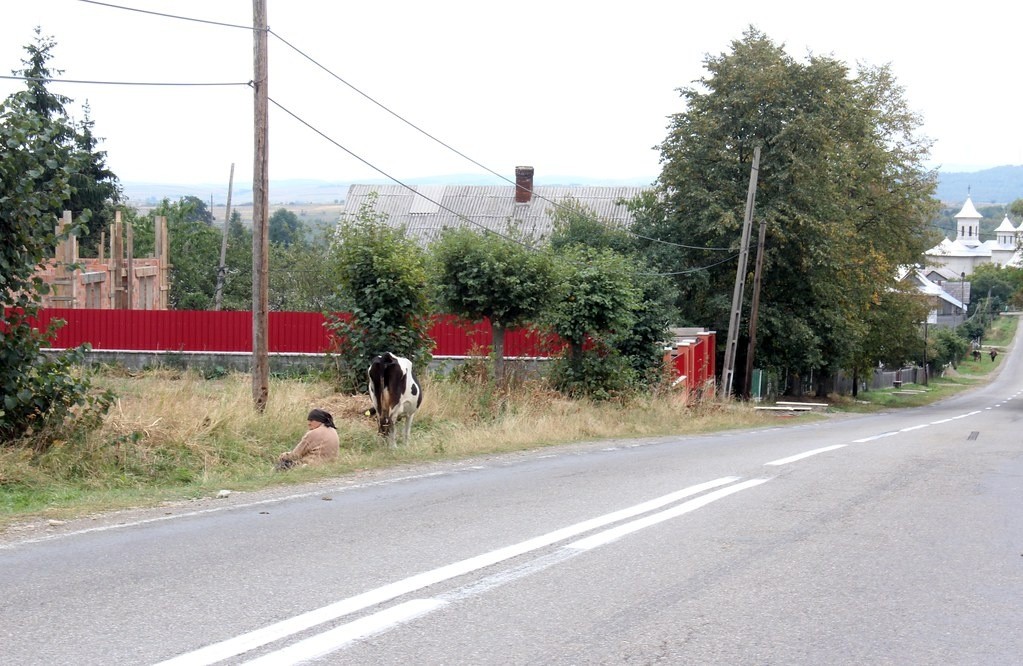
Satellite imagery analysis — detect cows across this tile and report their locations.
[363,351,423,450]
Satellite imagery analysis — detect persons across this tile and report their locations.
[989,348,998,363]
[270,409,339,471]
